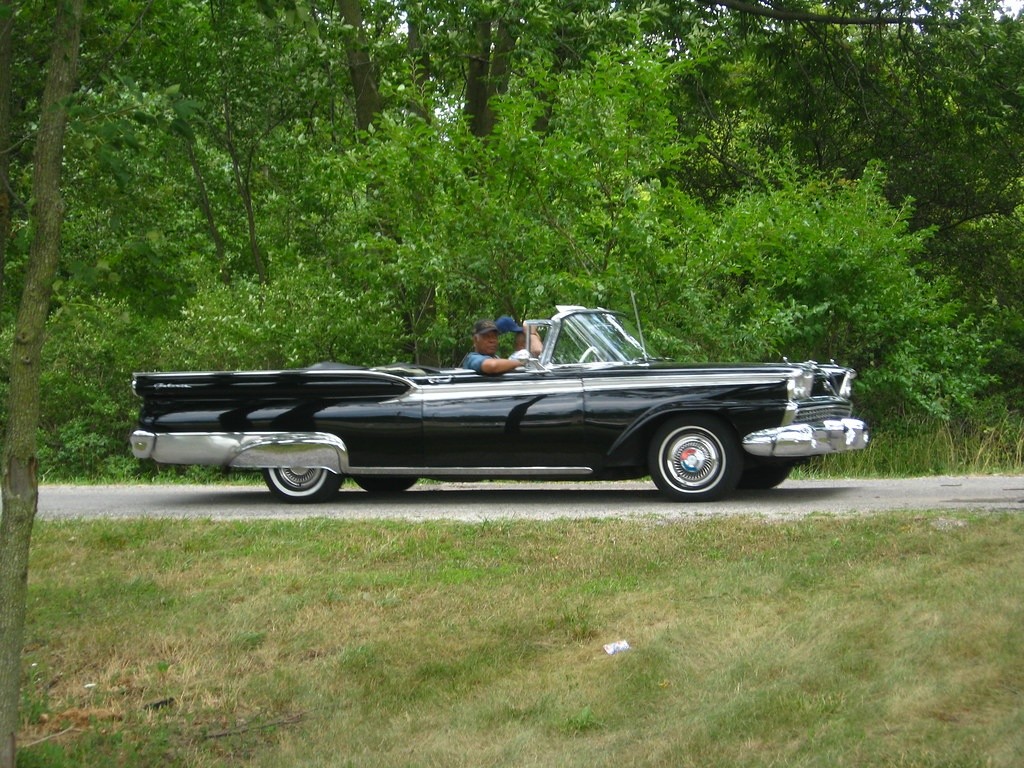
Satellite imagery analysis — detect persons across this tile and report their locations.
[509,319,544,367]
[462,320,524,375]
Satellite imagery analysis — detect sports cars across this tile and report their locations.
[126,302,872,501]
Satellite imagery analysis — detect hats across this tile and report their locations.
[473,320,501,336]
[494,316,523,335]
